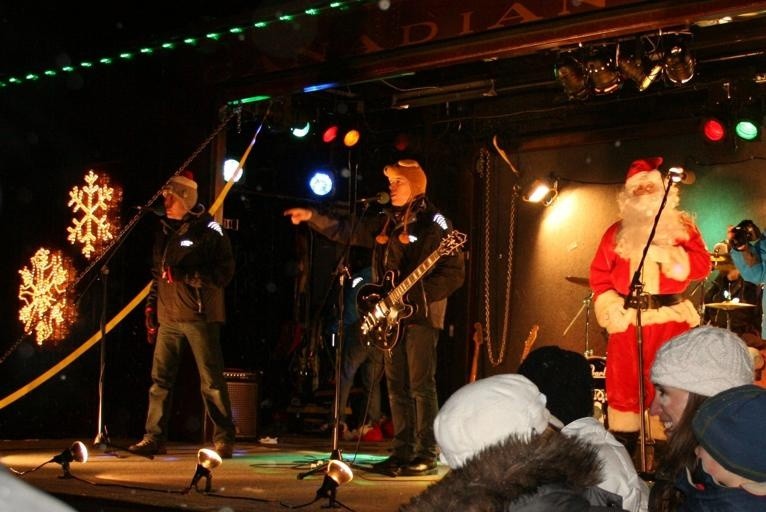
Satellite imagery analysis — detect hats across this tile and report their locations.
[651,325,754,397]
[515,345,595,426]
[693,384,766,482]
[709,241,728,261]
[625,156,663,188]
[162,170,198,209]
[383,160,427,198]
[433,374,564,471]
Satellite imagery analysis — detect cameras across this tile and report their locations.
[731,226,753,247]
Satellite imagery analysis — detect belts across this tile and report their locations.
[621,293,686,311]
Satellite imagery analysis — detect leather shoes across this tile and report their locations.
[372,457,437,475]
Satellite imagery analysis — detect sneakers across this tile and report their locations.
[215,441,232,458]
[129,437,166,455]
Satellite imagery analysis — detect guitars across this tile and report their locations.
[356,230,467,351]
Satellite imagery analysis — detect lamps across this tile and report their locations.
[553,38,696,102]
[316,109,360,148]
[516,173,560,206]
[695,76,763,142]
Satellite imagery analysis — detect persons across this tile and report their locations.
[703,219,766,341]
[127,172,235,459]
[517,346,642,512]
[399,374,623,512]
[315,245,387,441]
[647,326,766,512]
[589,157,711,483]
[280,158,466,478]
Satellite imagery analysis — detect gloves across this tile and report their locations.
[162,265,185,285]
[643,245,668,264]
[606,305,632,331]
[144,307,157,344]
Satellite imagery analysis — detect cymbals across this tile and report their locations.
[704,302,755,311]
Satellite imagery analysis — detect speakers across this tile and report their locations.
[203,371,258,441]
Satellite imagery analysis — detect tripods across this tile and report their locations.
[297,203,396,482]
[72,218,156,460]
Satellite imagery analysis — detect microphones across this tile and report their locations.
[355,191,391,204]
[673,169,696,185]
[137,204,165,216]
